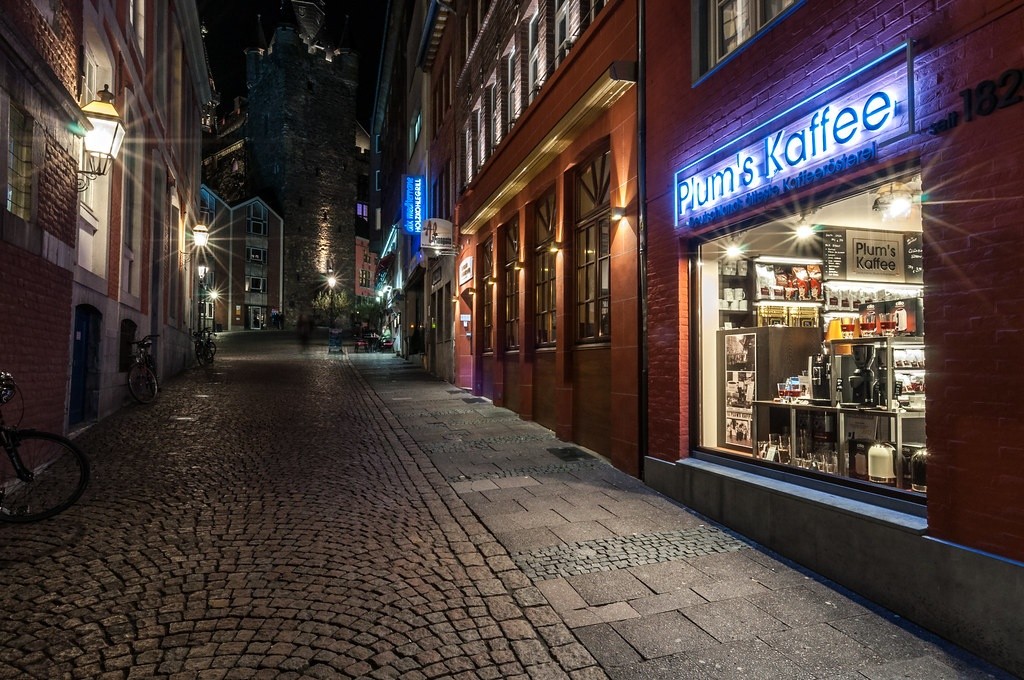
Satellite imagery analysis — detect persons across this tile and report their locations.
[377,326,392,351]
[270,310,284,330]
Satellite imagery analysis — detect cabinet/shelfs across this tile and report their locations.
[718,273,751,316]
[822,309,924,396]
[747,254,825,327]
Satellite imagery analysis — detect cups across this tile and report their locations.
[777,378,801,404]
[885,399,899,408]
[859,315,877,338]
[718,288,748,311]
[879,313,896,337]
[841,318,855,338]
[758,429,838,474]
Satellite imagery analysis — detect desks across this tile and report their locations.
[830,335,924,412]
[752,400,925,488]
[365,337,379,353]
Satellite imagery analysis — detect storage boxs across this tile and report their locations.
[858,302,885,337]
[885,297,923,337]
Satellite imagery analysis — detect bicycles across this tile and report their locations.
[194,326,218,366]
[0,372,89,524]
[125,334,163,403]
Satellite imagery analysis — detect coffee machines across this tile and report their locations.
[848,345,876,405]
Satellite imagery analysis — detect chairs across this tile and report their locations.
[352,334,370,353]
[381,338,396,353]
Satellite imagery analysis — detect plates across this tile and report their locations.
[902,406,925,412]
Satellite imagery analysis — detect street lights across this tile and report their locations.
[328,276,338,328]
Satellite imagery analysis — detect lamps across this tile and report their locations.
[488,277,496,285]
[514,261,523,271]
[468,288,475,295]
[550,241,562,253]
[78,84,127,193]
[179,218,210,271]
[611,207,626,220]
